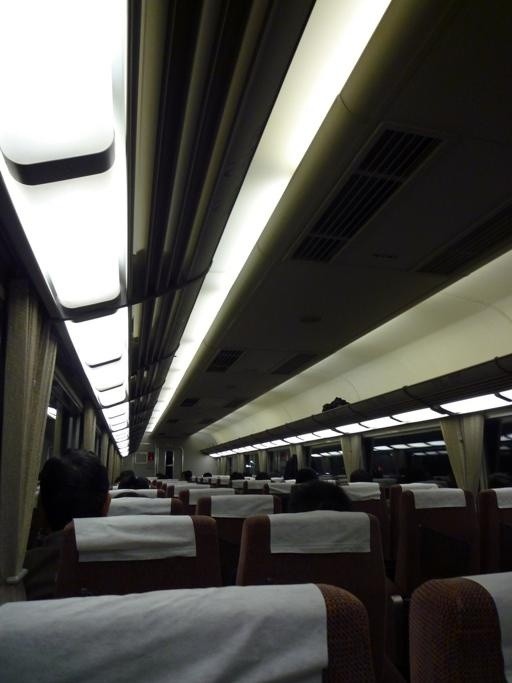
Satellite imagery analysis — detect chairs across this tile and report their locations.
[0,474,512,683]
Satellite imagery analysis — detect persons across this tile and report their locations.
[19,449,112,604]
[487,469,511,489]
[294,467,320,484]
[350,468,378,482]
[394,465,428,484]
[289,481,352,510]
[118,464,272,491]
